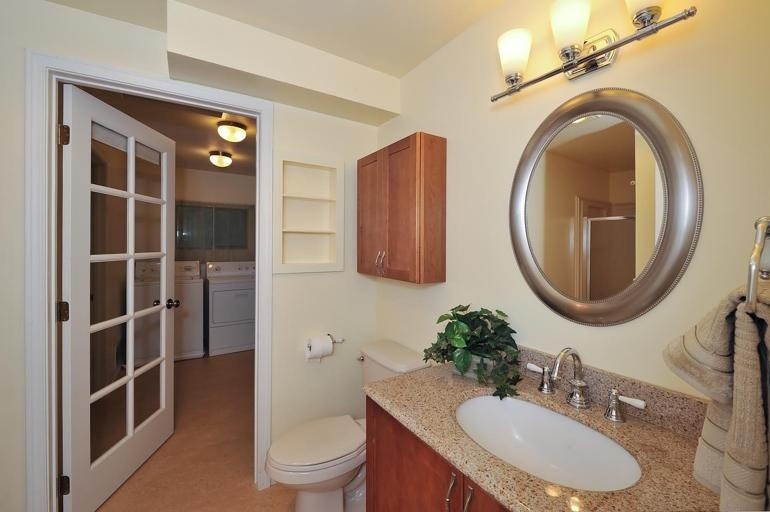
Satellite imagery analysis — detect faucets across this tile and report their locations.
[547,346,591,412]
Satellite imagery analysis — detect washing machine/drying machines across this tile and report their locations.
[205,261,255,356]
[134,260,206,361]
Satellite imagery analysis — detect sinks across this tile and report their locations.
[449,385,646,495]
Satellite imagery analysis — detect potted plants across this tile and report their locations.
[424,304,523,399]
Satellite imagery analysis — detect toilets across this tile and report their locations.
[263,338,433,512]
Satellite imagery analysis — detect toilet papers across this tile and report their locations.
[303,333,333,365]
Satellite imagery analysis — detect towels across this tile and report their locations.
[662,277,770,512]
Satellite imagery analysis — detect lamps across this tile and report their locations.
[491,0,697,101]
[209,151,233,168]
[509,87,705,327]
[217,121,247,143]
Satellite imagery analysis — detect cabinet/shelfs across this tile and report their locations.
[273,154,345,272]
[365,394,512,512]
[357,132,447,285]
